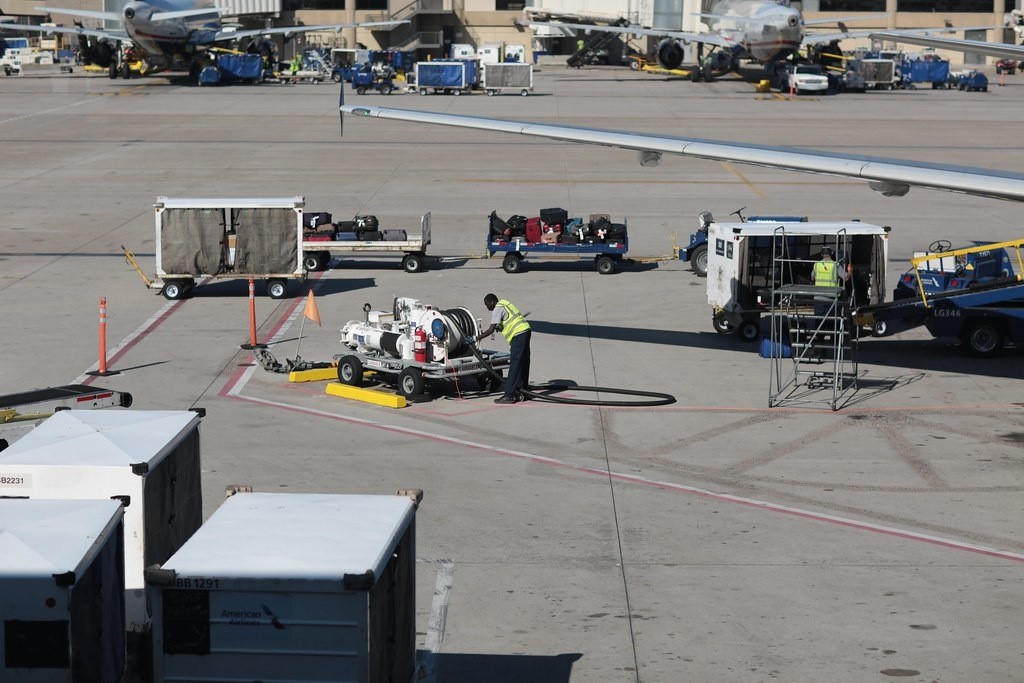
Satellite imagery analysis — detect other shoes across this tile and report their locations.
[523,394,528,401]
[494,396,515,404]
[808,352,827,359]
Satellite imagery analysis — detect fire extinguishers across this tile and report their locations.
[414,325,426,363]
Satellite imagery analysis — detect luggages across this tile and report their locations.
[303,212,407,242]
[492,208,625,244]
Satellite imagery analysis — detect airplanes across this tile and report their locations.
[866,30,1024,62]
[513,0,1009,89]
[0,0,412,84]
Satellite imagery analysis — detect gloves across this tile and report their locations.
[463,335,477,345]
[495,324,503,332]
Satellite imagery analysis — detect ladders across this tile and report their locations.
[767,226,858,412]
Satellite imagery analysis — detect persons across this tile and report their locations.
[810,247,853,342]
[576,40,584,49]
[295,51,303,70]
[474,294,532,405]
[290,57,299,84]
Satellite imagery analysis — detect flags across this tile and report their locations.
[303,290,320,325]
